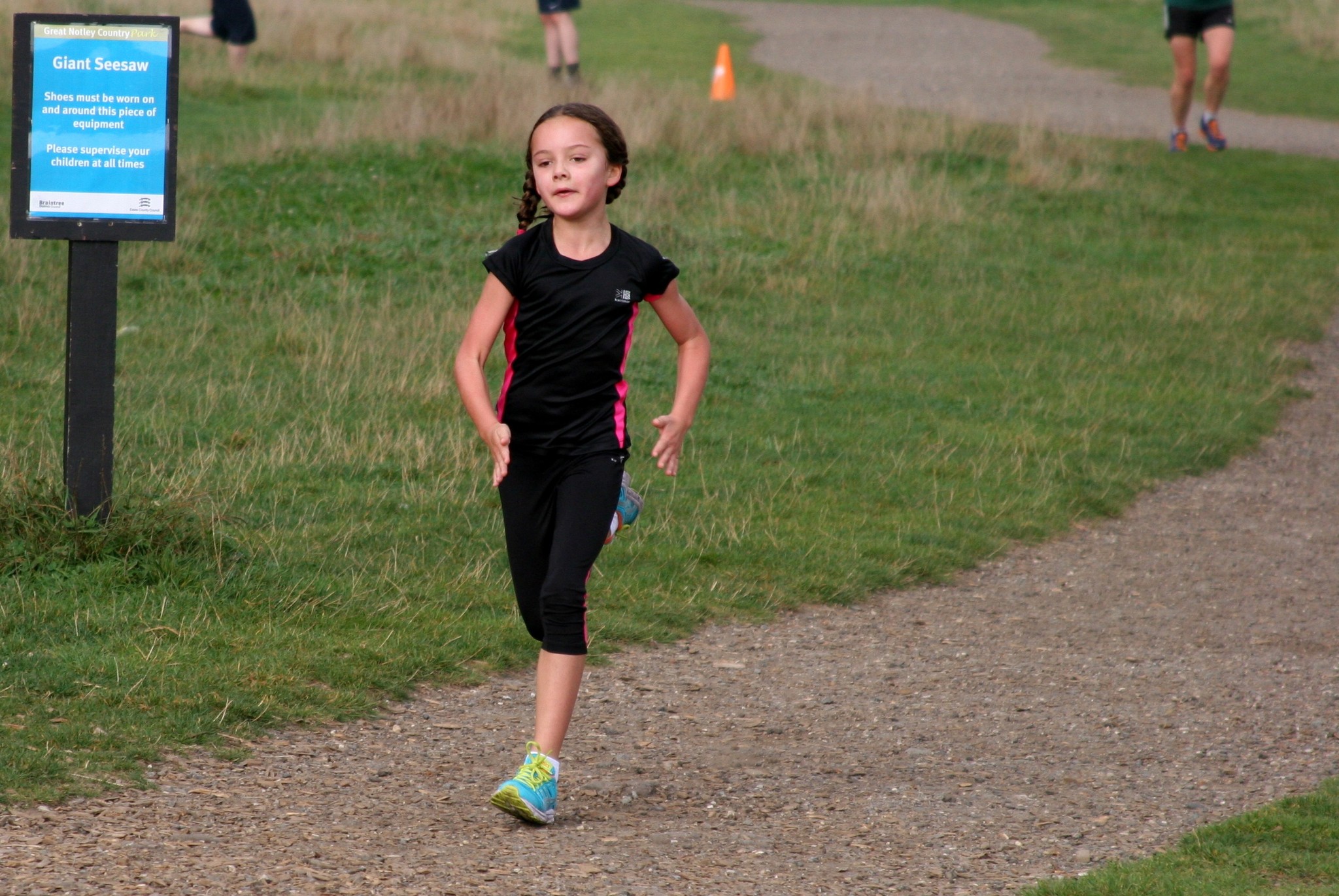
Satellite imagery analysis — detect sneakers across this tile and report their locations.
[1170,127,1187,154]
[1198,117,1227,152]
[615,470,644,538]
[490,741,558,825]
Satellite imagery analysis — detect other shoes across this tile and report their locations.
[548,66,562,84]
[567,64,581,84]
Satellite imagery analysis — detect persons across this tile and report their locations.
[451,104,710,827]
[532,0,583,85]
[1161,0,1238,153]
[154,0,257,81]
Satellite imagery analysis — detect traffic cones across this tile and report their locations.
[710,44,737,103]
